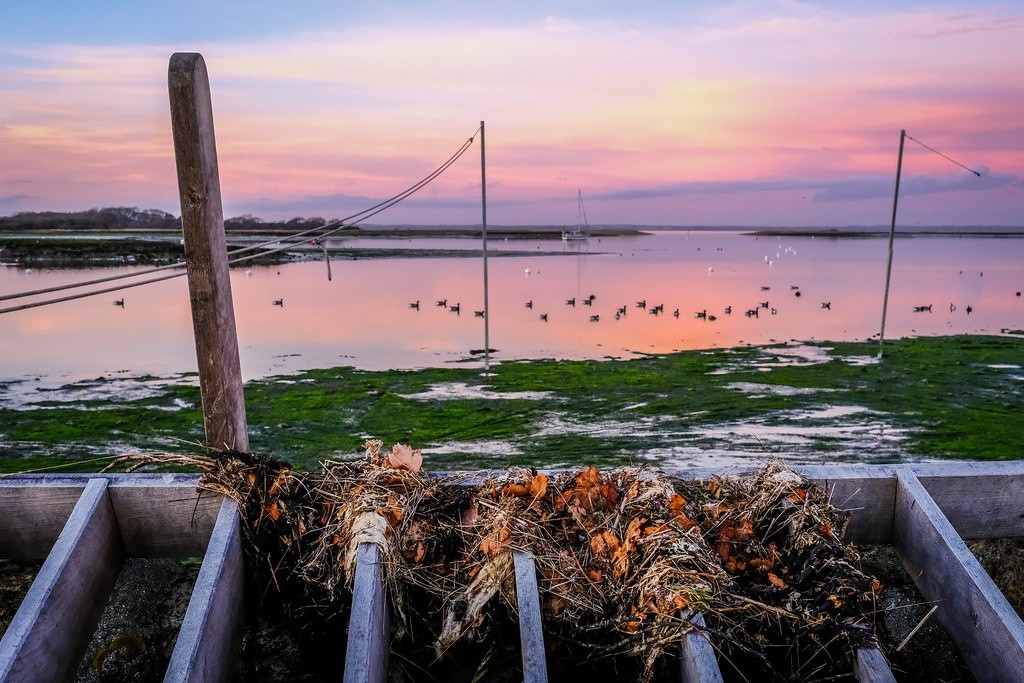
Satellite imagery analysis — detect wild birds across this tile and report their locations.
[615,305,627,316]
[947,302,972,309]
[959,270,1021,294]
[636,300,664,312]
[474,311,485,314]
[759,286,777,311]
[565,295,596,302]
[24,234,821,277]
[526,301,532,305]
[724,306,759,314]
[912,304,933,309]
[113,298,125,303]
[790,285,831,306]
[541,314,547,317]
[272,299,284,303]
[409,301,419,306]
[437,300,447,304]
[672,308,718,319]
[591,315,599,319]
[450,302,460,309]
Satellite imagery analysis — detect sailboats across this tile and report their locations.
[560,189,591,241]
